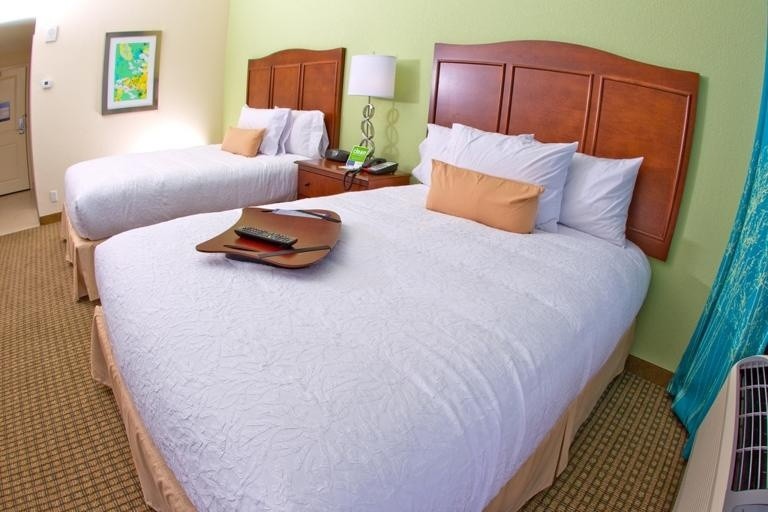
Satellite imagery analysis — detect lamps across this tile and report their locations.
[347,49,396,161]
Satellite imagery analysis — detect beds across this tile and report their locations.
[86,38,700,511]
[57,45,346,302]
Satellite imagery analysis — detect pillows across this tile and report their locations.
[442,120,579,233]
[424,158,544,234]
[514,132,646,249]
[219,126,268,158]
[273,104,329,159]
[410,122,536,188]
[236,103,295,155]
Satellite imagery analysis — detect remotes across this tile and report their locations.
[234,224,298,246]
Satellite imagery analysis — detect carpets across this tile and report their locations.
[0,221,687,512]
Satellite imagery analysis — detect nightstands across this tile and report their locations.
[293,158,411,199]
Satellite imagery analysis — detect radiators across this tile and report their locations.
[669,352,766,511]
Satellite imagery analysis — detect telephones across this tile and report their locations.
[359,158,399,175]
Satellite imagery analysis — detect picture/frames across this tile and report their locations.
[100,30,162,116]
